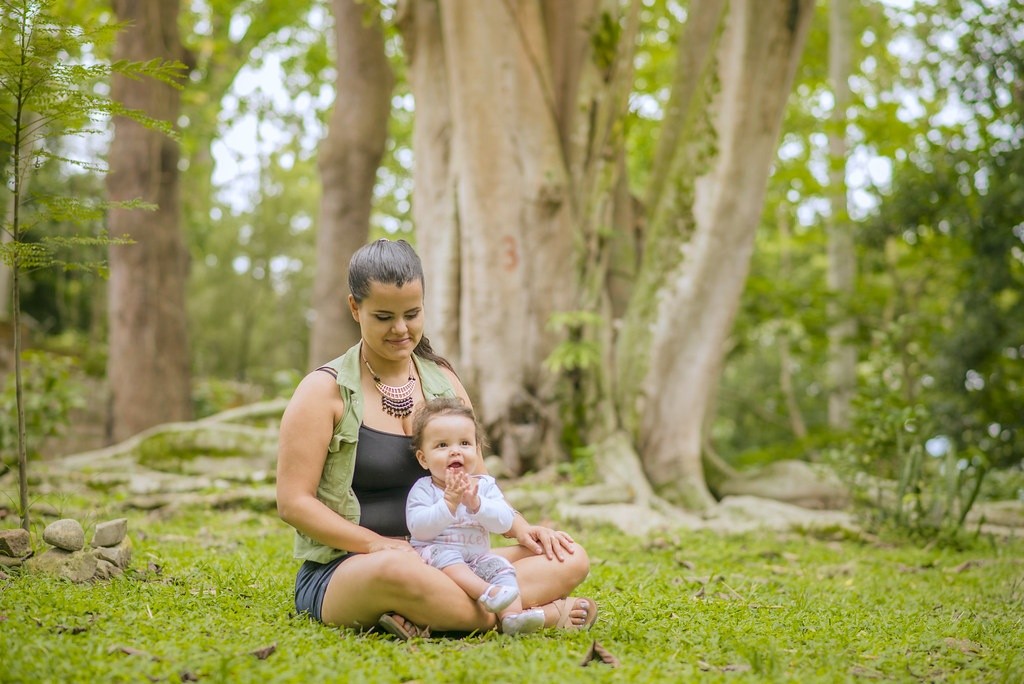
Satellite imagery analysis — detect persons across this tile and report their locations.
[276,238,597,640]
[406,397,546,635]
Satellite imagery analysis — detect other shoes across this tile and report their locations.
[501,609,545,634]
[479,584,519,613]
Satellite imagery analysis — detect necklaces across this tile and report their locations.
[361,349,416,418]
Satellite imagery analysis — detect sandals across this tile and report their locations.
[544,597,597,632]
[379,613,420,641]
